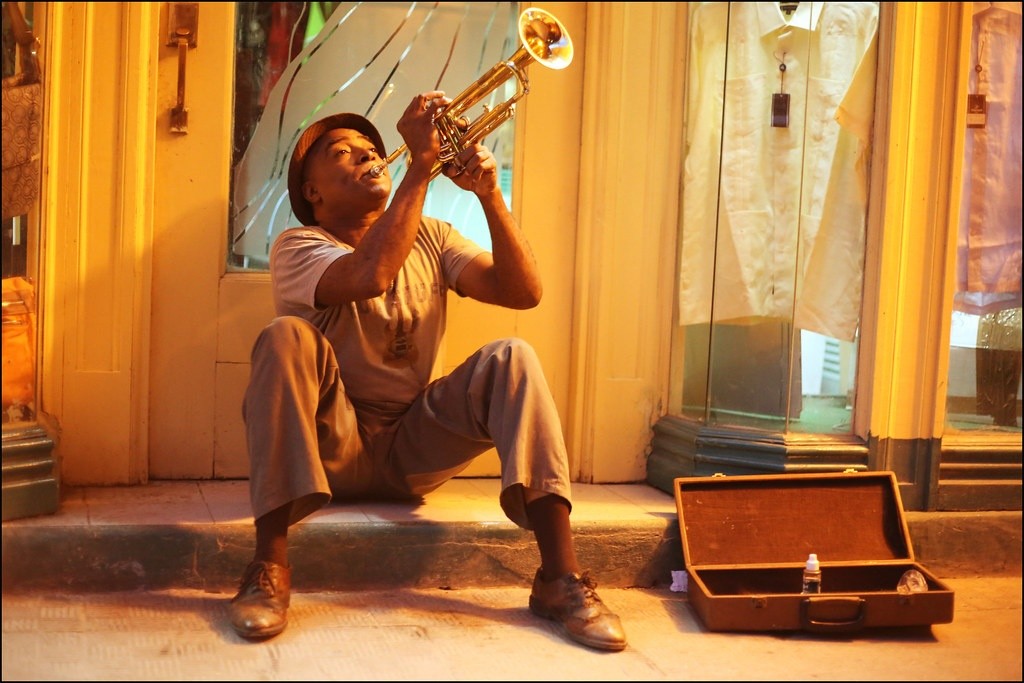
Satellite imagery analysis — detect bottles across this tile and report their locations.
[802,554,821,595]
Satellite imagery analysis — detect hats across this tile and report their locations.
[288,112,386,227]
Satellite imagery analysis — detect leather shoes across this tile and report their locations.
[529,566,628,650]
[227,560,292,637]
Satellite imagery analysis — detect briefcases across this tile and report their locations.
[673,470,955,633]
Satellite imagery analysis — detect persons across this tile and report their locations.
[228,90,630,652]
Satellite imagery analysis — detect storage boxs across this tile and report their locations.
[673,471,954,632]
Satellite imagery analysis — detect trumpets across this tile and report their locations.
[369,8,575,176]
